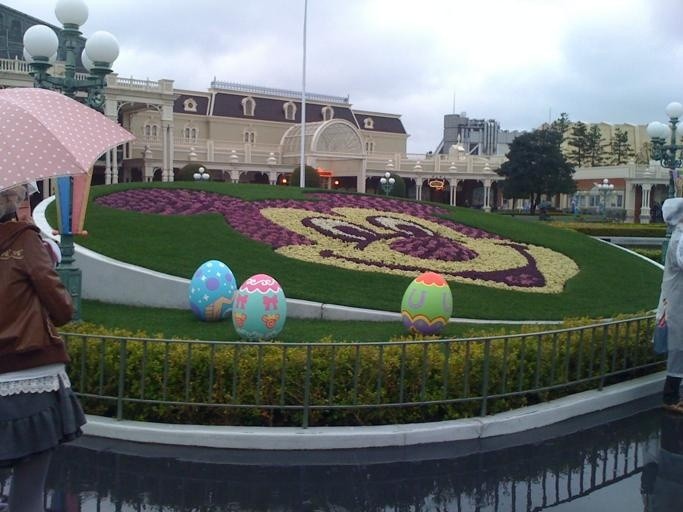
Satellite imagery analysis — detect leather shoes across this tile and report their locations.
[661,396,683,411]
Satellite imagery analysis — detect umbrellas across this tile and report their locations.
[0,87,139,195]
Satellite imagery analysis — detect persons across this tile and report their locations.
[463,199,472,208]
[660,200,664,221]
[0,186,88,512]
[525,194,577,214]
[653,197,683,414]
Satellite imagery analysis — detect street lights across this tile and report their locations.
[596,177,614,224]
[22,0,119,326]
[379,172,396,197]
[192,167,210,181]
[646,102,682,264]
